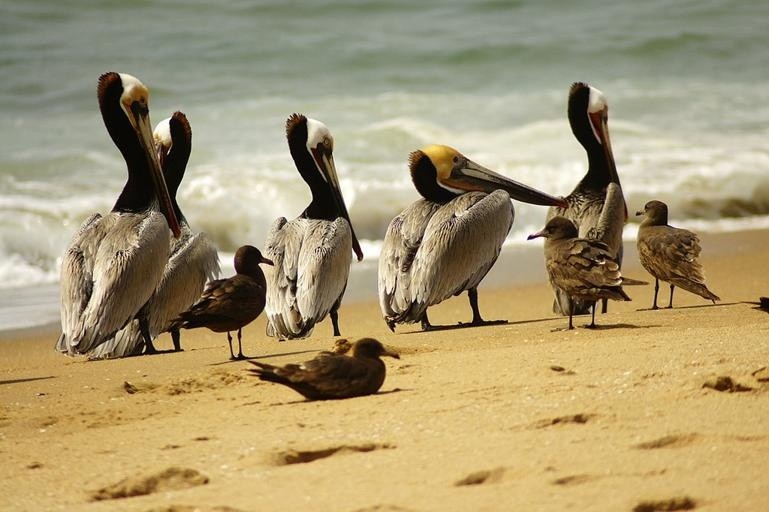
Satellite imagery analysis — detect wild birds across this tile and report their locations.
[157,245,275,360]
[376,143,570,333]
[526,216,650,333]
[53,71,182,357]
[545,82,629,317]
[635,200,721,312]
[83,110,223,361]
[241,337,401,400]
[259,113,364,342]
